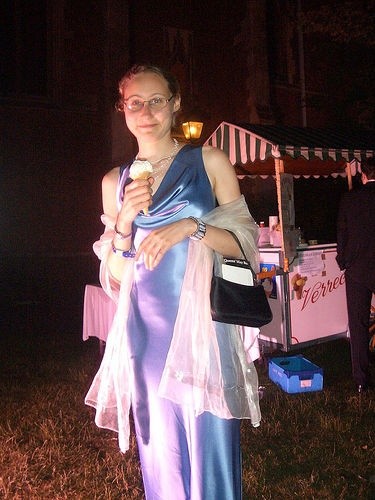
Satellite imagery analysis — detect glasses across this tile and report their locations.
[123,93,174,113]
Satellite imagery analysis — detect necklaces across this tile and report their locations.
[139,137,179,181]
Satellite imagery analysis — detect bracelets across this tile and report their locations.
[113,223,133,241]
[111,240,136,258]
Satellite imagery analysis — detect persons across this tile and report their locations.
[334,156,375,392]
[83,60,263,500]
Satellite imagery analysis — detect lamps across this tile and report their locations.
[183,116,205,142]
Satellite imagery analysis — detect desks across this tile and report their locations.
[82,283,117,354]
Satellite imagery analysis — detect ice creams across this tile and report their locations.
[292,272,307,299]
[129,160,153,215]
[262,278,273,298]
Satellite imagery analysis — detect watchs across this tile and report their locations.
[187,216,207,241]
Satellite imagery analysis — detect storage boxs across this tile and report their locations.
[268,353,324,393]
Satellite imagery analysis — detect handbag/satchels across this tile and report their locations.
[210,228,274,328]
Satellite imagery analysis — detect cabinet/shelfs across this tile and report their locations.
[256,243,349,352]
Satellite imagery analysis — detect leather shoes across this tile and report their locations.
[357,384,369,393]
